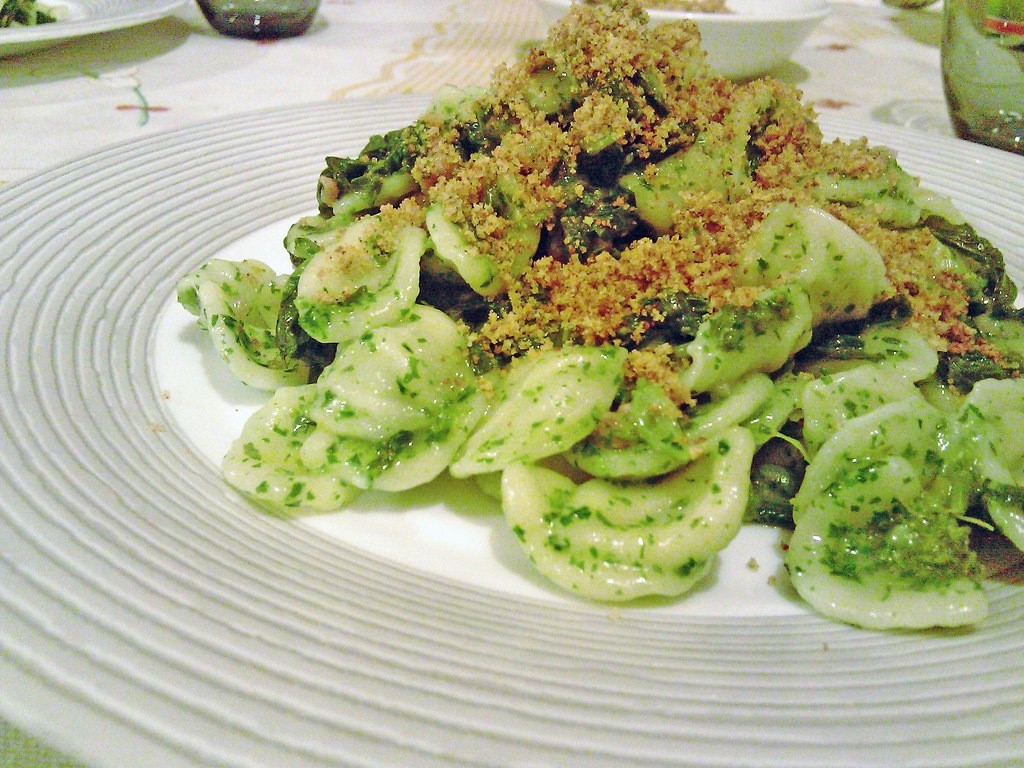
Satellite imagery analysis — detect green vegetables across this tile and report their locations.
[0,0,57,29]
[275,67,1024,530]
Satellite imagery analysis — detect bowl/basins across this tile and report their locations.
[539,0,832,83]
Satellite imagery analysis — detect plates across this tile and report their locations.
[0,0,192,57]
[0,99,1024,768]
